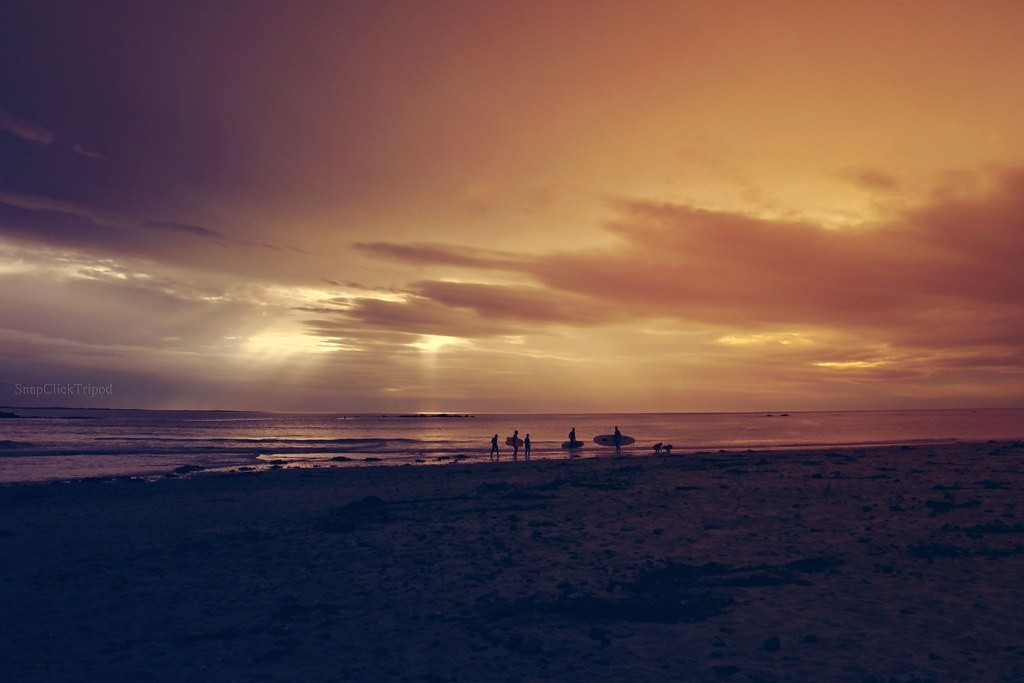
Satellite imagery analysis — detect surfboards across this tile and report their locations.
[594,435,635,446]
[505,436,524,447]
[562,440,584,448]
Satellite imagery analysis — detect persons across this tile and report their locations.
[569,427,576,443]
[524,433,531,458]
[614,426,621,453]
[662,445,673,453]
[653,442,663,453]
[512,430,518,459]
[490,434,499,459]
